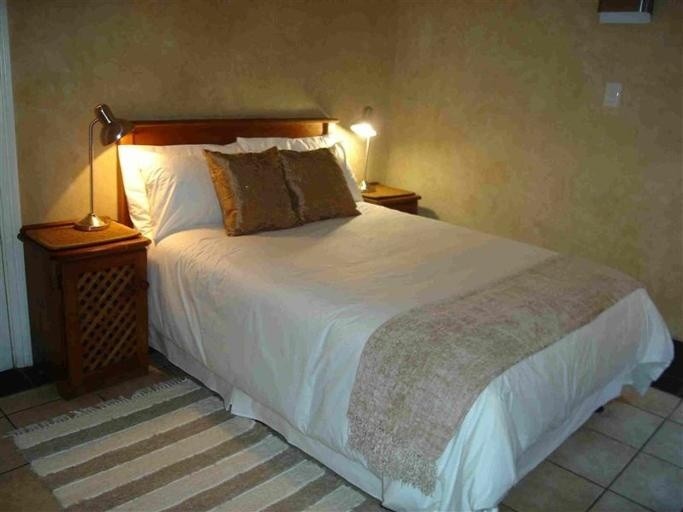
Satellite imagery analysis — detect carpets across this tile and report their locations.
[17,377,387,512]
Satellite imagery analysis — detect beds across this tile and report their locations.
[114,113,676,512]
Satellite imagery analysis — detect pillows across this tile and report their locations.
[117,135,364,235]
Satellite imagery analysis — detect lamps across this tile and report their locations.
[68,99,134,234]
[351,102,386,192]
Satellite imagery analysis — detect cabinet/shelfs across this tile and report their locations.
[15,217,153,402]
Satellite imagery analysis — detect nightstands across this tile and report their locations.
[364,182,423,215]
